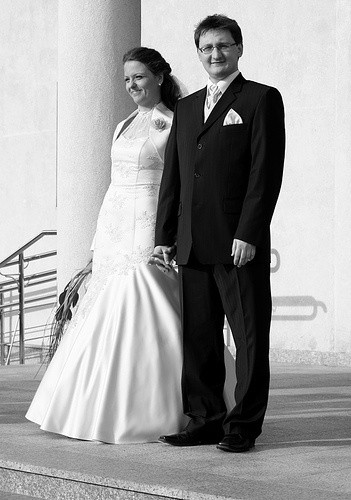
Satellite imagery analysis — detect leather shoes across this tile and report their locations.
[158,430,224,446]
[216,437,255,453]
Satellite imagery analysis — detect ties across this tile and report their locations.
[207,86,221,115]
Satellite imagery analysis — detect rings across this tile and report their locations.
[246,257,251,262]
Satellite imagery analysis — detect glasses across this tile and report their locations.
[198,42,238,54]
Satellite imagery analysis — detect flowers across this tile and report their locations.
[153,118,167,131]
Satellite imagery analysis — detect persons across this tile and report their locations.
[25,47,237,445]
[147,15,286,453]
